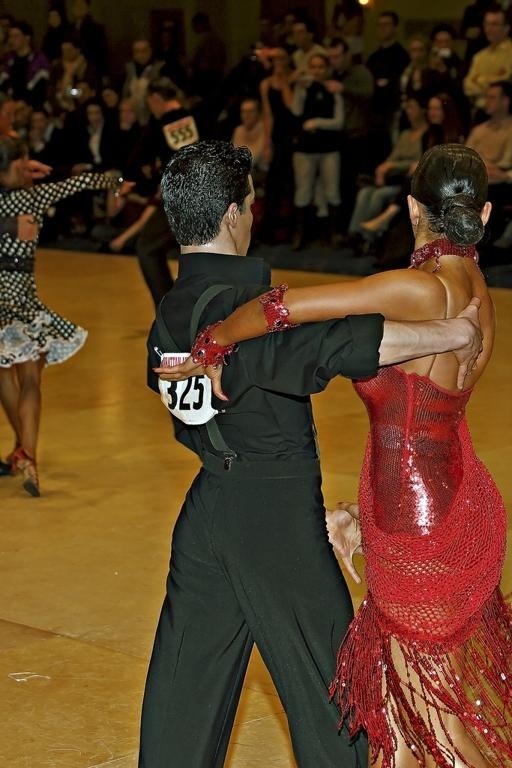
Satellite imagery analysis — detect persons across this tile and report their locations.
[0,213,39,241]
[1,2,512,282]
[1,134,138,500]
[139,75,214,311]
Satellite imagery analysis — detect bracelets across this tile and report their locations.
[188,320,238,370]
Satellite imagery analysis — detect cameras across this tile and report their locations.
[64,88,82,97]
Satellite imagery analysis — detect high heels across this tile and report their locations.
[7,446,41,499]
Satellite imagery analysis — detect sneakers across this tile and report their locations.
[0,443,22,475]
